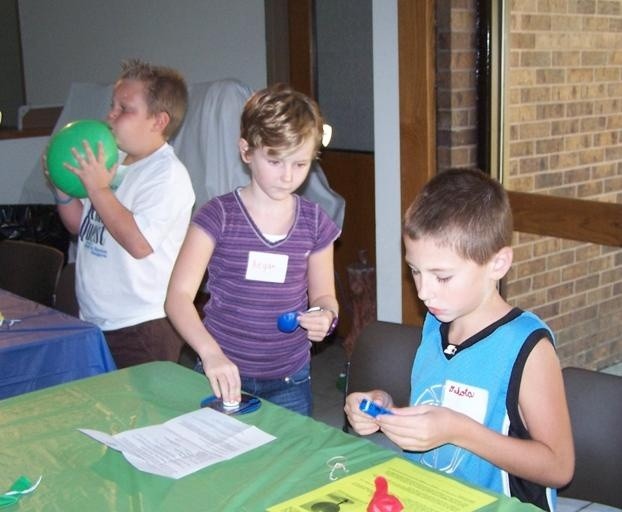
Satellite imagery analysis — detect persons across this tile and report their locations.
[343,167,576,511]
[163,81,343,419]
[40,58,196,370]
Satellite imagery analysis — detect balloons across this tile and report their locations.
[0,475,32,509]
[45,120,122,201]
[367,475,403,512]
[276,312,305,333]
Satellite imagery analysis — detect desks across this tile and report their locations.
[0,360,540,512]
[2,291,116,398]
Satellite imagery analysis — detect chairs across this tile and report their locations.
[1,239,64,308]
[345,321,421,451]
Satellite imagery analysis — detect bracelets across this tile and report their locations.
[319,307,339,337]
[53,197,72,205]
